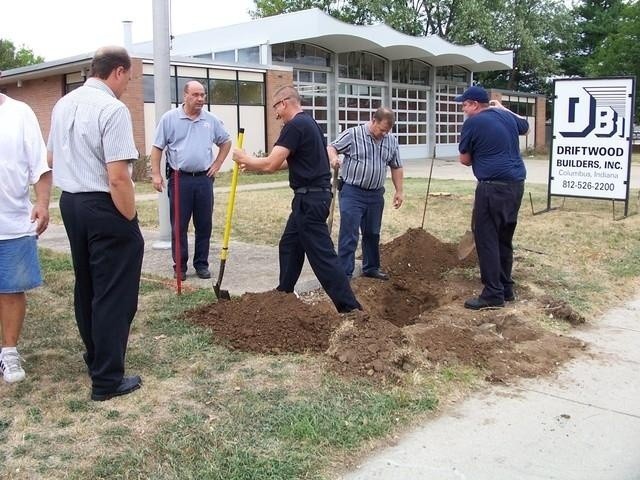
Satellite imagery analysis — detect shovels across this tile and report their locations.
[457,102,496,260]
[212,128,246,300]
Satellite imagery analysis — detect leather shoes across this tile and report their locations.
[196,269,210,279]
[465,294,514,310]
[364,271,389,280]
[174,272,186,281]
[92,375,142,401]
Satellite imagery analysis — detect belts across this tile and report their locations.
[294,187,330,194]
[480,179,524,185]
[169,166,210,176]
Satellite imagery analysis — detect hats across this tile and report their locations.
[453,86,489,103]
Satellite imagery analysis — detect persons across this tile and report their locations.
[454,86,529,310]
[326,106,404,284]
[0,69,53,383]
[233,84,365,313]
[45,43,146,401]
[150,80,231,281]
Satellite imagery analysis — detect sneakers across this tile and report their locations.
[0,352,26,383]
